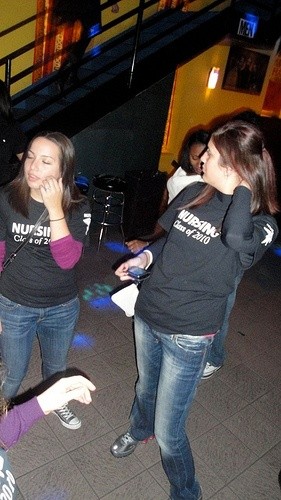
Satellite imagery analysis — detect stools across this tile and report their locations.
[91,178,126,249]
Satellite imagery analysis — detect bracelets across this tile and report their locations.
[48,217,65,222]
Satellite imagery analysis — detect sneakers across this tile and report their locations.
[200,362,223,379]
[68,74,78,83]
[52,77,63,97]
[166,485,203,500]
[110,431,155,458]
[51,402,81,430]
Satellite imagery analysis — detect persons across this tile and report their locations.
[1,131,85,431]
[1,322,96,499]
[125,123,245,379]
[111,120,278,500]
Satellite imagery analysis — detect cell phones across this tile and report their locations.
[125,266,150,281]
